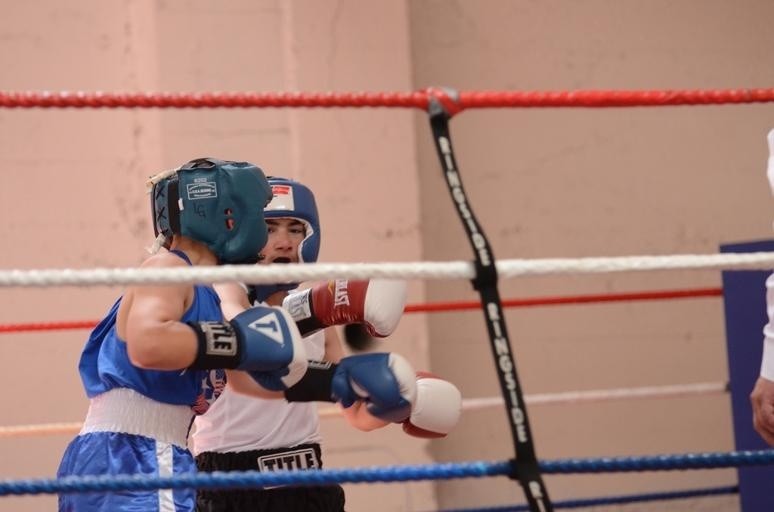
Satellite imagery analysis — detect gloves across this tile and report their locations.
[402,372,461,439]
[185,306,309,392]
[284,351,417,421]
[280,278,404,337]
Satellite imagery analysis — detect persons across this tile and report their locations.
[179,169,464,512]
[47,154,421,510]
[747,272,772,452]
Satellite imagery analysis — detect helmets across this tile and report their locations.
[261,175,322,263]
[146,159,272,260]
